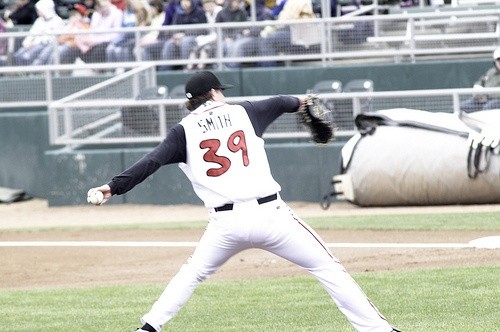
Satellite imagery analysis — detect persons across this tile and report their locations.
[87,71,402,332]
[452,47,500,113]
[0,0,454,76]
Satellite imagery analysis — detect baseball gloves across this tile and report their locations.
[296,96,339,147]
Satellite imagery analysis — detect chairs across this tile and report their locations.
[296,78,374,127]
[0,19,370,68]
[122,84,190,133]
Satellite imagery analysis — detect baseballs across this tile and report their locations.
[89,190,104,205]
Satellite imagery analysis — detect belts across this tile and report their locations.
[209,191,279,213]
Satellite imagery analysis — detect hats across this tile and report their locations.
[185,71,234,99]
